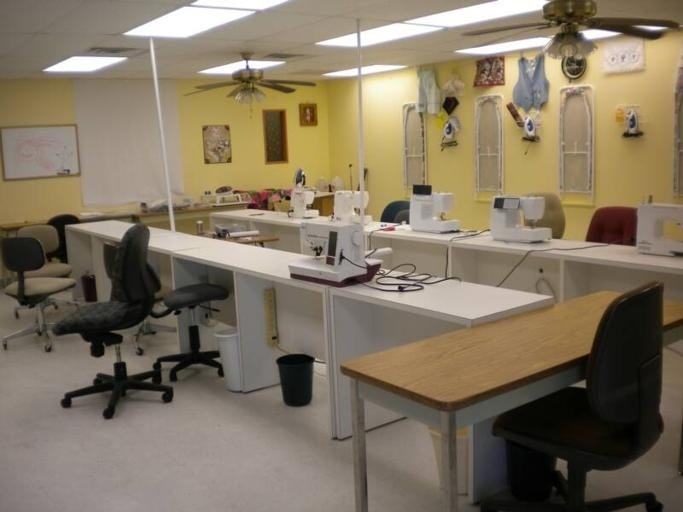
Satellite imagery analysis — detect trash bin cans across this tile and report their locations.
[213,328,241,391]
[275,354,315,407]
[80,274,97,302]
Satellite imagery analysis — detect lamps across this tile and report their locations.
[229,82,266,105]
[539,25,598,61]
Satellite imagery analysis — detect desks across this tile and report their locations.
[339,288,683,509]
[64,205,682,439]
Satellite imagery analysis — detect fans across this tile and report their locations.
[184,50,316,95]
[462,1,679,49]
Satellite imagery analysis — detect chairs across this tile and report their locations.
[523,194,568,238]
[0,235,79,350]
[488,281,664,512]
[10,225,78,324]
[379,199,411,225]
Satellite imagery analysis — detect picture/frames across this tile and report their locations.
[298,103,318,126]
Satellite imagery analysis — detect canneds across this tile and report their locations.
[197,221,204,235]
